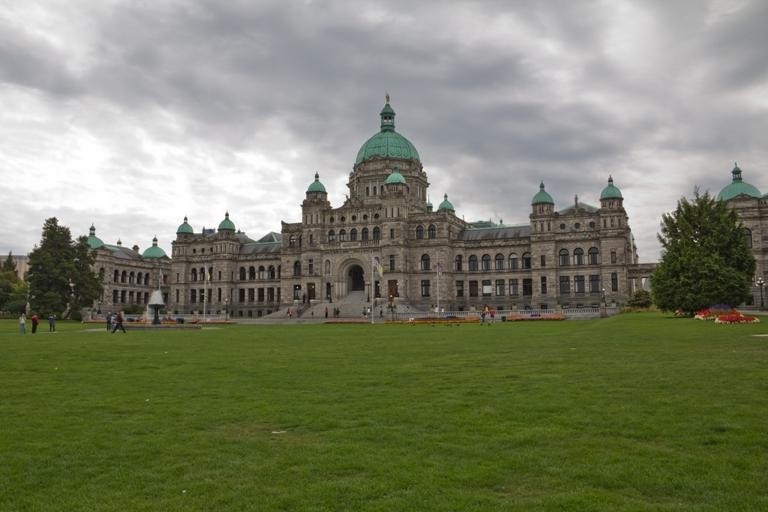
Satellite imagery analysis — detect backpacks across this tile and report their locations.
[116,314,126,322]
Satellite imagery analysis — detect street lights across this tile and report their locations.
[756,277,766,308]
[602,288,606,303]
[225,298,228,320]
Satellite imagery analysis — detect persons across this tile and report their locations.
[31,313,40,333]
[101,311,126,333]
[18,311,27,336]
[50,313,55,332]
[285,292,517,326]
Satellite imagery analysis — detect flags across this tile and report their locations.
[158,268,165,287]
[204,264,211,282]
[438,262,445,277]
[373,257,384,278]
